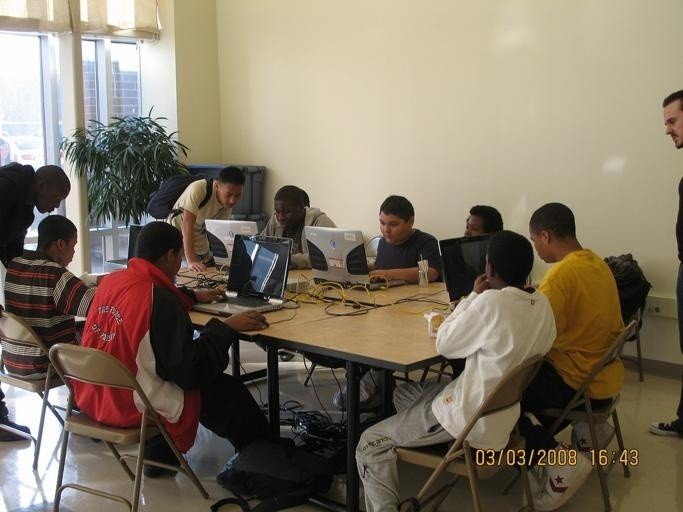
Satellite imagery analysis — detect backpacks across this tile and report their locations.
[146,174,213,225]
[604,253,653,341]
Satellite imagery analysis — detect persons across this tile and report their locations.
[515,202,628,511]
[164,166,246,342]
[0,161,70,442]
[450,205,504,381]
[254,185,337,362]
[648,89,681,438]
[68,222,271,480]
[333,195,442,416]
[355,228,557,511]
[4,214,97,413]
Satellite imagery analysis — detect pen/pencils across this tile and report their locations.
[201,256,208,263]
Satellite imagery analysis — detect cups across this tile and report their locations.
[417,259,429,288]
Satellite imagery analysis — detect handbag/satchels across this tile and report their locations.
[210,438,334,512]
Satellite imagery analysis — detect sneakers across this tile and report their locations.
[571,419,615,459]
[533,441,593,512]
[649,422,679,436]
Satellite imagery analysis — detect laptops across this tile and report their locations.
[204,218,259,272]
[190,233,292,316]
[89,224,144,287]
[439,234,535,311]
[304,226,407,290]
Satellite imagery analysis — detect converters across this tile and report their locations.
[287,279,307,291]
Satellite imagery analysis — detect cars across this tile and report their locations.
[0,134,44,172]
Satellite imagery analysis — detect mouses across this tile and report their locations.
[256,319,269,328]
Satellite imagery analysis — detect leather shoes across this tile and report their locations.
[0,421,30,441]
